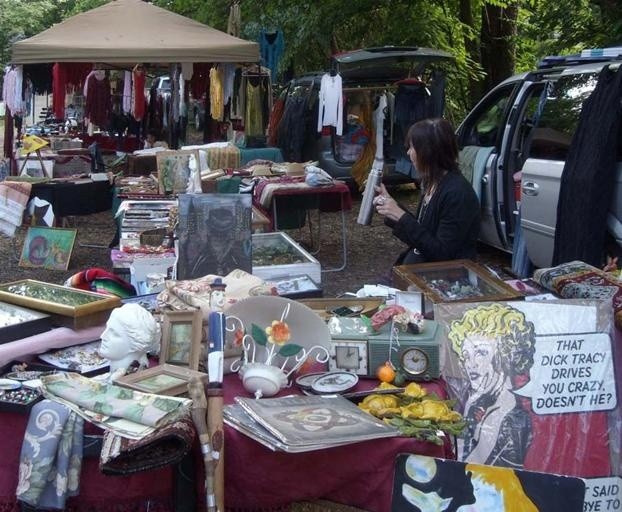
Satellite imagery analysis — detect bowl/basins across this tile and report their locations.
[140,229,175,253]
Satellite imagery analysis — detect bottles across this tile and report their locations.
[357,167,385,226]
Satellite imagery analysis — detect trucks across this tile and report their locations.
[188,89,206,130]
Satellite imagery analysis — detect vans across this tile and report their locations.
[267,44,459,192]
[447,48,622,274]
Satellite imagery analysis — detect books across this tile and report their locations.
[112,198,179,254]
[220,391,401,454]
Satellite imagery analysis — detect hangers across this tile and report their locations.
[322,61,342,78]
[210,62,223,72]
[241,64,270,77]
[88,65,109,78]
[132,64,148,74]
[350,85,397,92]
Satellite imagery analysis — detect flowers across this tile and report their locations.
[229,320,305,356]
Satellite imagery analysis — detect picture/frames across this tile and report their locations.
[113,362,206,396]
[159,310,202,370]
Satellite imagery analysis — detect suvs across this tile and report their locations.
[150,75,172,101]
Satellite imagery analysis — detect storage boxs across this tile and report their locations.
[252,232,322,294]
[0,278,122,331]
[1,301,53,344]
[392,259,525,322]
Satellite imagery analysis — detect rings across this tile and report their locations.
[377,198,384,206]
[381,196,387,201]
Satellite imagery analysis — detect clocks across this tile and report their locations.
[400,348,429,377]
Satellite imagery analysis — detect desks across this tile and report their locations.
[246,161,351,270]
[6,173,119,251]
[0,375,454,512]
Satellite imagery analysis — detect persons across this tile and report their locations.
[207,278,226,390]
[98,303,162,376]
[448,306,536,470]
[190,208,248,277]
[372,117,481,265]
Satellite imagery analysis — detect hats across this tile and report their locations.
[206,209,236,233]
[281,163,304,177]
[240,166,284,177]
